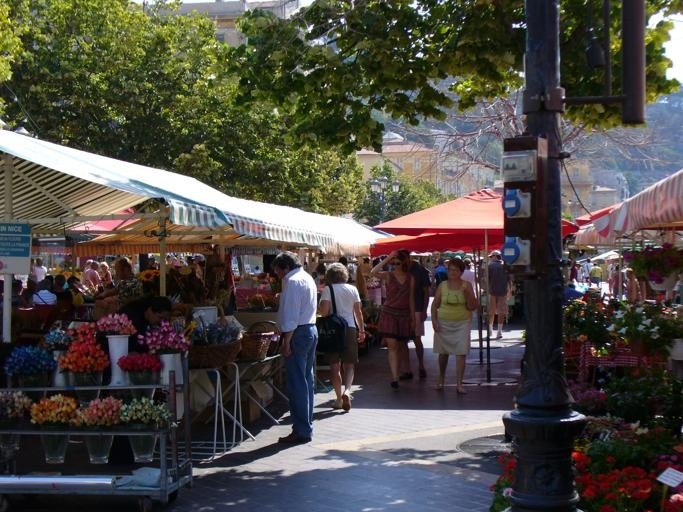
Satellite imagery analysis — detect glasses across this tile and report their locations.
[391,262,400,266]
[447,292,459,305]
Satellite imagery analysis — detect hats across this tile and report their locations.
[488,250,501,255]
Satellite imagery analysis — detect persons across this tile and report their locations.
[672,270,683,305]
[164,254,206,285]
[315,249,527,393]
[560,258,642,301]
[110,296,171,356]
[0,254,157,327]
[272,250,320,445]
[318,262,365,412]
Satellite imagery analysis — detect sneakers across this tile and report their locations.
[280,432,306,442]
[497,332,502,338]
[331,390,351,411]
[456,386,465,393]
[434,383,443,390]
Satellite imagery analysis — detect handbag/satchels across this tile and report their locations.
[316,314,348,353]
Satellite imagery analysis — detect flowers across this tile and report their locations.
[96,313,137,336]
[137,319,190,354]
[30,394,81,424]
[119,396,175,427]
[66,323,97,343]
[0,388,33,422]
[42,324,72,350]
[58,337,111,374]
[69,396,123,426]
[4,345,58,377]
[117,354,164,374]
[138,270,160,283]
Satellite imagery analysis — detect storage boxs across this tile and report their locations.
[213,360,274,424]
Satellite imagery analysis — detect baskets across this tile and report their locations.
[239,334,272,362]
[196,338,243,368]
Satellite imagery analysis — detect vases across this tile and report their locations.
[83,435,114,465]
[41,436,70,464]
[159,352,185,421]
[5,376,16,388]
[104,334,131,387]
[128,372,156,400]
[17,376,48,404]
[51,350,76,387]
[76,370,103,407]
[128,427,158,463]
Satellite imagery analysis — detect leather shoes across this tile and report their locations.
[391,368,426,391]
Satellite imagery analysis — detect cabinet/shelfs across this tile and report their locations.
[0,384,194,503]
